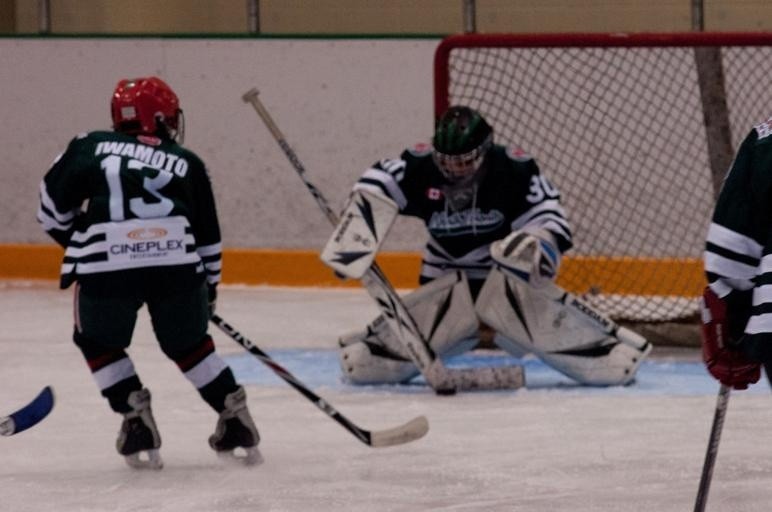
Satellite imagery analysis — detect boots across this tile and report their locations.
[114,388,162,456]
[203,386,262,452]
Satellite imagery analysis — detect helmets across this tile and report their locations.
[426,103,494,166]
[108,76,180,138]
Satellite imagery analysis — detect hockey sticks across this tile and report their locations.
[208,313,429,448]
[0,384,54,437]
[241,86,524,394]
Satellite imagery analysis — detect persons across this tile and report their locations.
[315,102,657,388]
[695,109,772,392]
[36,74,262,456]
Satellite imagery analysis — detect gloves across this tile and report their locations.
[698,279,764,392]
